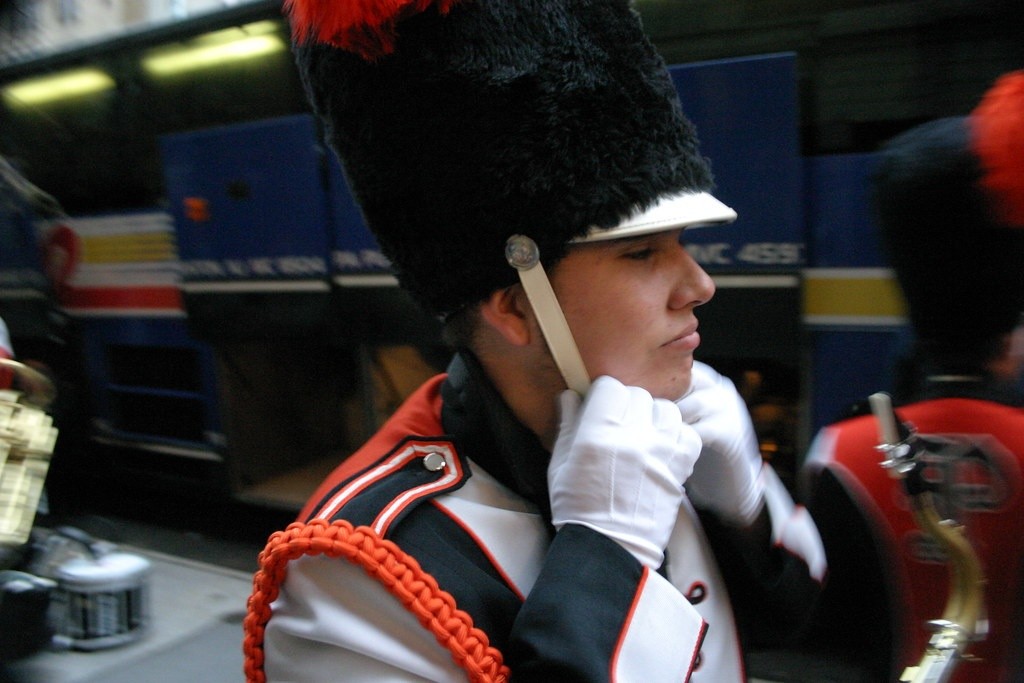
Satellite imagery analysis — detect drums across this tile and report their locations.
[55,552,154,651]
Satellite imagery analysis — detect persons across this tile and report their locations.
[241,188,854,682]
[796,321,1024,683]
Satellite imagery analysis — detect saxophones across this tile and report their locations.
[870,390,990,682]
[0,357,59,546]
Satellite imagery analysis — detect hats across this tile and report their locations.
[868,69,1024,393]
[284,1,740,341]
